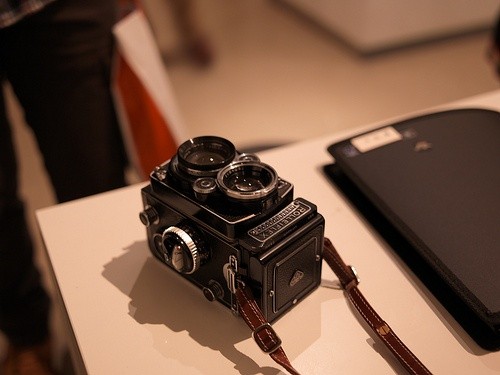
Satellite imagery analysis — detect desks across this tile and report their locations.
[33,91,500,375]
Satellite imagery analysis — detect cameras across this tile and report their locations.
[141,136,326,327]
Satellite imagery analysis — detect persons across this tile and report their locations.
[1,1,135,375]
